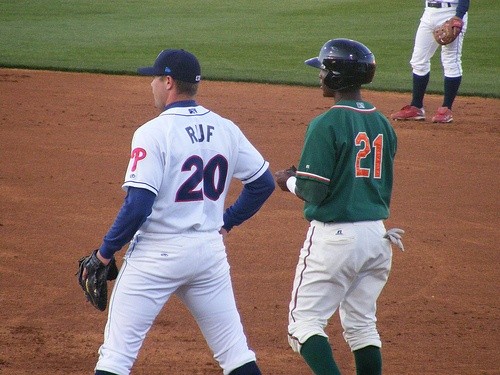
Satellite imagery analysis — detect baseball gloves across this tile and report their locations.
[77,248,117,312]
[432,15,464,45]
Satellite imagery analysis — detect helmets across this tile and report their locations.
[305,38,376,90]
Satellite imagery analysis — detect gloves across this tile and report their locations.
[384,229,406,251]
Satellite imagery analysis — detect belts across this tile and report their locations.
[428,2,451,8]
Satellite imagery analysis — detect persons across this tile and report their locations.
[275,38,397,374]
[75,48,276,375]
[391,0,470,123]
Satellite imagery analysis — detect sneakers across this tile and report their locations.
[391,104,425,121]
[432,106,453,123]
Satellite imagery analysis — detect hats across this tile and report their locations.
[137,49,200,83]
